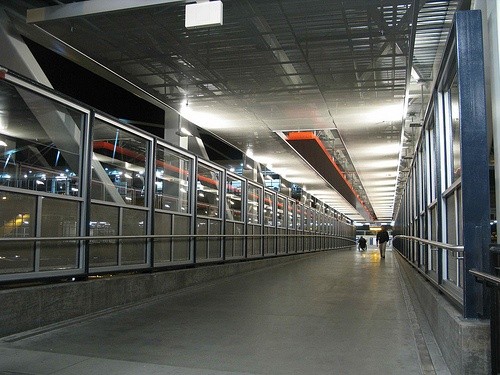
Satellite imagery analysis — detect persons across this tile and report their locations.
[376,225,389,259]
[357,236,367,252]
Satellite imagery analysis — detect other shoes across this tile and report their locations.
[382,255,385,259]
